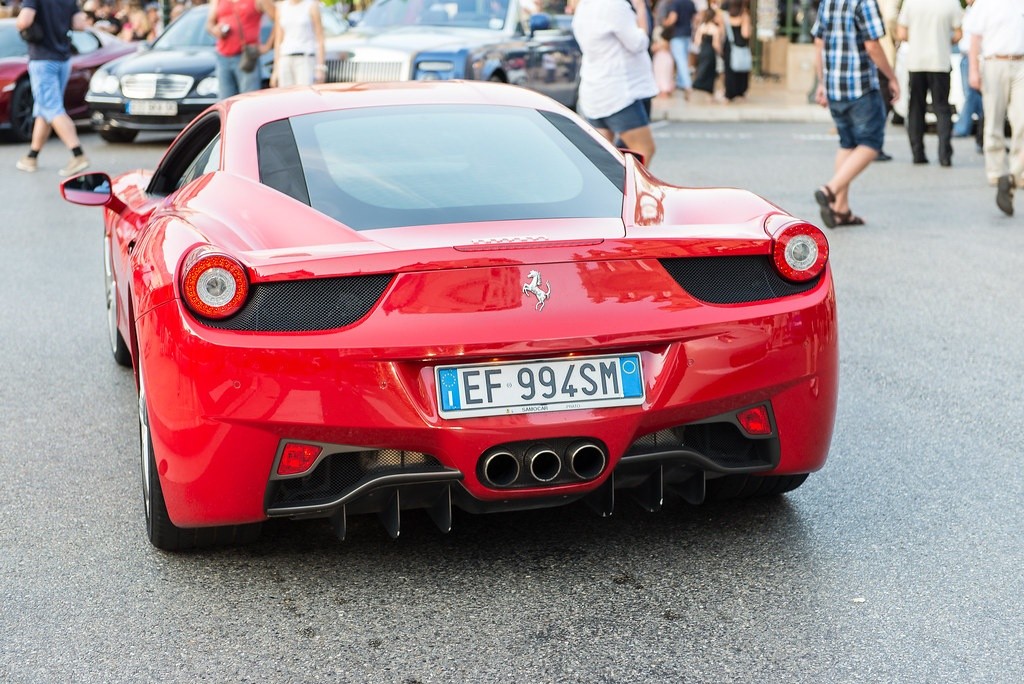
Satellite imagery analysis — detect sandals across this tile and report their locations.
[814,185,837,228]
[995,173,1017,215]
[835,209,865,223]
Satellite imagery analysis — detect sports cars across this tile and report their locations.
[60,78,838,550]
[0,17,151,142]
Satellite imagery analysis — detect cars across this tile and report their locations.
[81,5,350,142]
[333,0,583,112]
[890,31,980,126]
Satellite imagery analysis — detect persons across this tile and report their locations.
[204,0,329,104]
[809,0,900,228]
[0,0,203,179]
[572,0,1024,218]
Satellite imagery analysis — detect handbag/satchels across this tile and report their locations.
[728,43,752,70]
[21,0,46,43]
[238,43,259,73]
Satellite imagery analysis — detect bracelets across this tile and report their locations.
[315,64,328,72]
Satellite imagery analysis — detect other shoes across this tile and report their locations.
[16,155,37,172]
[57,154,90,176]
